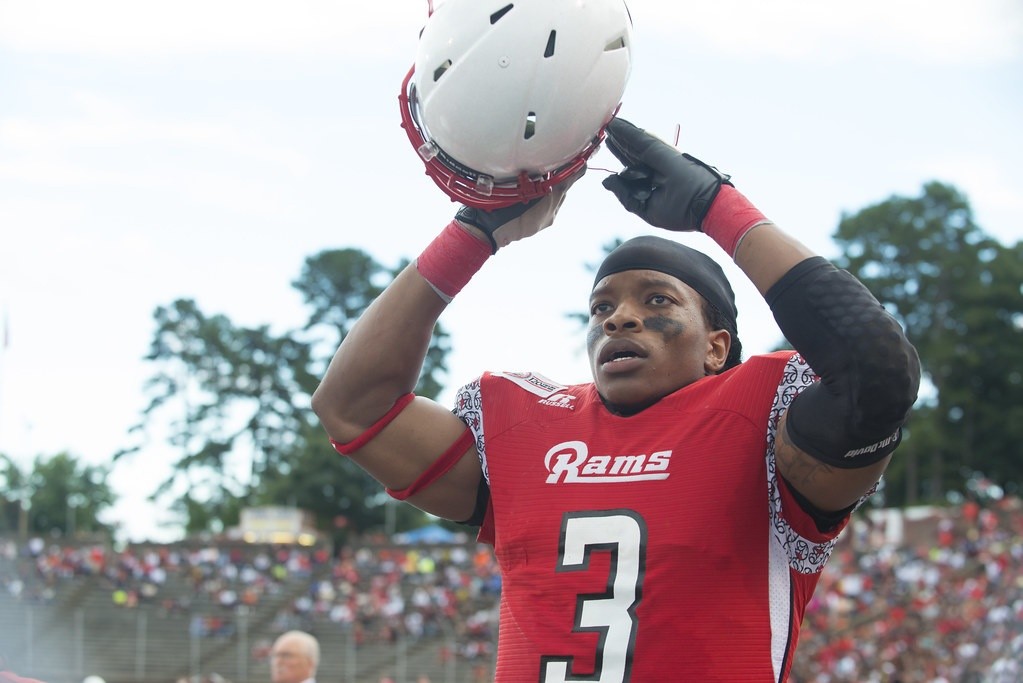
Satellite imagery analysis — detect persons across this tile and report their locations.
[0,538,502,661]
[785,495,1023,683]
[312,118,921,683]
[271,630,319,683]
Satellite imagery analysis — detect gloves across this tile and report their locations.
[454,160,588,255]
[603,108,735,235]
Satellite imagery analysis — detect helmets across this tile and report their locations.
[398,0,632,214]
